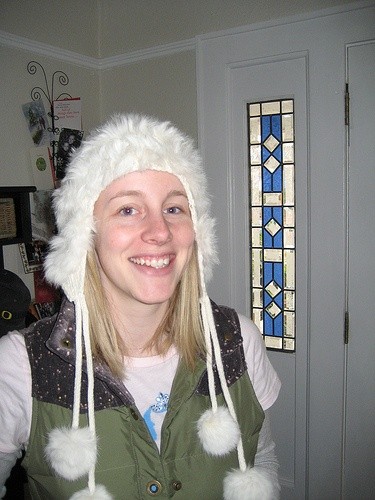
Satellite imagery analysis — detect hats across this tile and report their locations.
[42,113,276,500]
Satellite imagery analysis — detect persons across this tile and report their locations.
[0,109,286,500]
[56,133,79,180]
